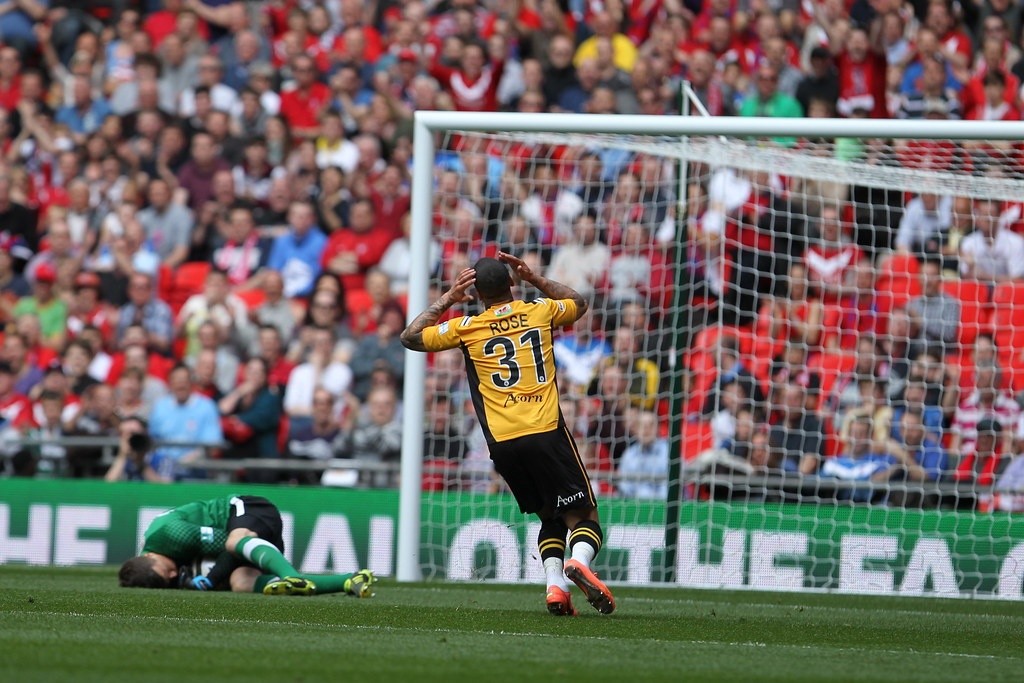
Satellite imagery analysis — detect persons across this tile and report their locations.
[400,252,617,616]
[118,494,375,599]
[0,0,1024,516]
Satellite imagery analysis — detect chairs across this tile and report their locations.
[688,255,1024,454]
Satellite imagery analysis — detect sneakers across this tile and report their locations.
[546,584,580,616]
[562,557,617,616]
[343,569,379,599]
[265,574,316,597]
